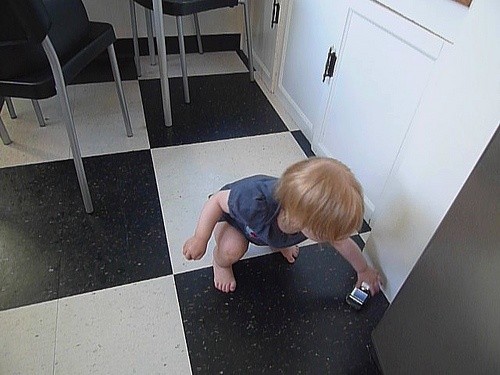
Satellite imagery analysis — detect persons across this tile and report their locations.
[183,156,385,297]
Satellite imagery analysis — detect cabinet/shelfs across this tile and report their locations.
[276,1,449,229]
[244,1,289,95]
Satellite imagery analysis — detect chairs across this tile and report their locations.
[0,1,133,215]
[129,0,256,104]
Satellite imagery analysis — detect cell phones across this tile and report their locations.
[346,283,371,311]
